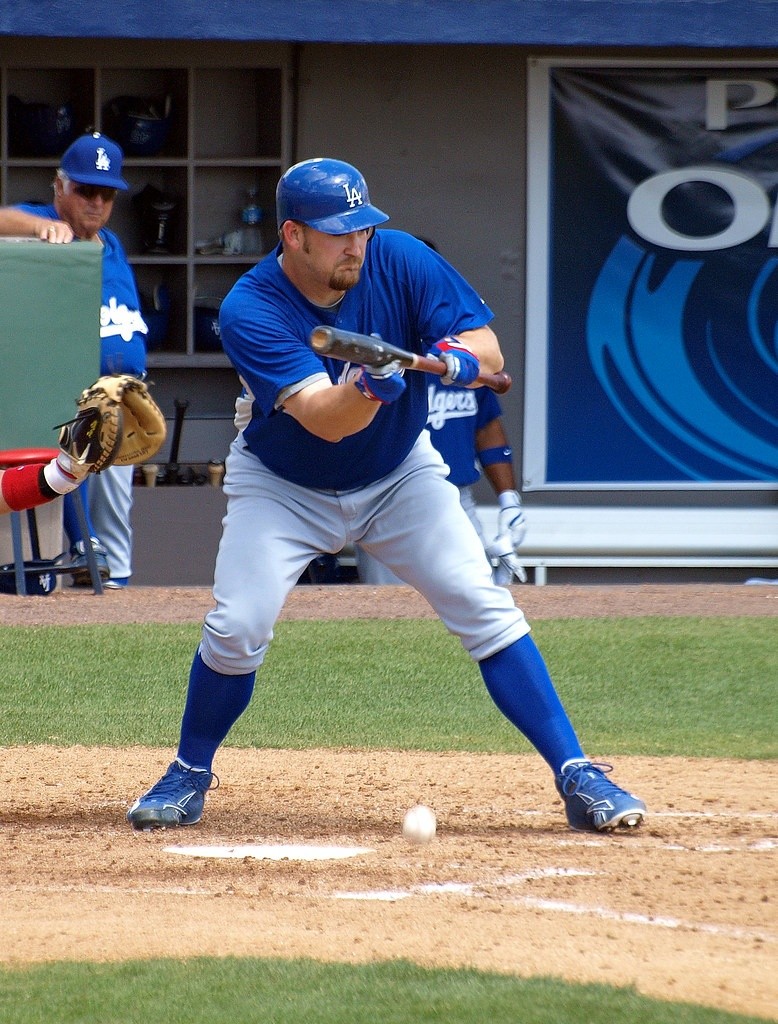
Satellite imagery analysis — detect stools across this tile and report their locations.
[0,448,105,597]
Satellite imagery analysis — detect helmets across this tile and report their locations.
[276,159,390,234]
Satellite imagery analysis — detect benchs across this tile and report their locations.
[334,503,778,586]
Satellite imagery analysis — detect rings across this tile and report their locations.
[48,228,54,235]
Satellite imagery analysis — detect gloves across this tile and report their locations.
[426,335,480,387]
[497,491,526,547]
[354,359,406,405]
[486,540,528,587]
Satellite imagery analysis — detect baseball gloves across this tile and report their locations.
[60,373,168,472]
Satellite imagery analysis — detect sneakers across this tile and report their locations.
[128,760,219,828]
[103,578,128,589]
[554,761,646,833]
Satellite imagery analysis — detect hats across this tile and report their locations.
[60,131,128,192]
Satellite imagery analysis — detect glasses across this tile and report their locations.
[70,181,118,202]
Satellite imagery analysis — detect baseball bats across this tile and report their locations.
[131,397,223,486]
[310,325,513,397]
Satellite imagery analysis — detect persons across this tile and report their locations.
[128,158,646,835]
[0,373,165,514]
[0,131,149,589]
[358,232,528,586]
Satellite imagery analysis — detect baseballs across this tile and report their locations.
[403,806,437,840]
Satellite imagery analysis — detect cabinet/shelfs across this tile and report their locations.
[0,36,292,370]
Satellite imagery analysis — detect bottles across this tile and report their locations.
[242,187,263,257]
